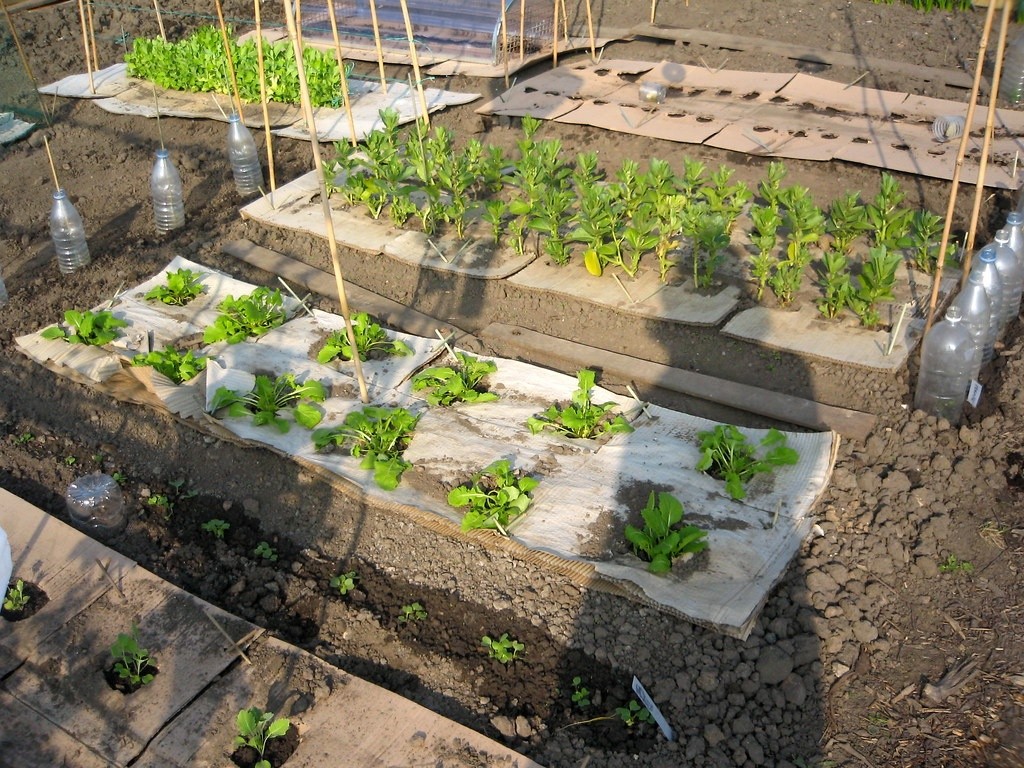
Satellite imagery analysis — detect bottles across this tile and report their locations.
[972,229,1019,343]
[227,113,264,198]
[151,149,186,235]
[964,247,1002,384]
[1003,211,1024,308]
[999,32,1023,103]
[933,114,968,142]
[0,273,8,305]
[51,189,91,275]
[638,82,667,105]
[914,306,970,427]
[952,269,991,393]
[65,470,127,539]
